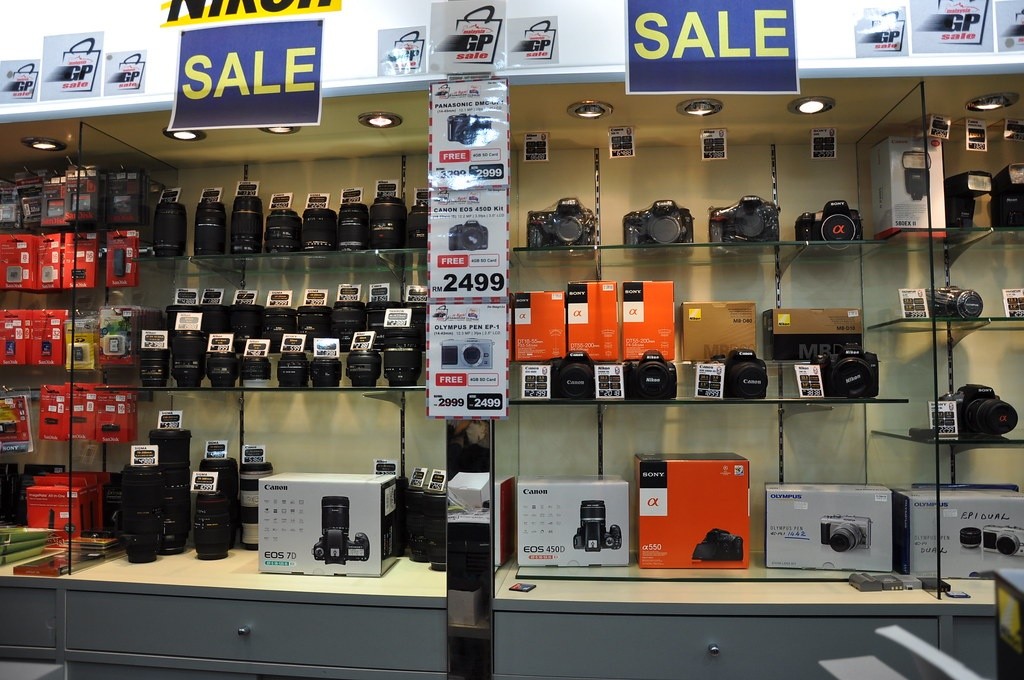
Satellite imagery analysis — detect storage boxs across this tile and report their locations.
[764,307,864,363]
[871,136,945,238]
[636,452,750,570]
[682,301,757,362]
[891,488,1024,583]
[515,477,630,567]
[514,279,676,361]
[765,483,893,572]
[259,472,398,578]
[0,461,110,538]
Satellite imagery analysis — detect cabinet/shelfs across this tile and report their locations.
[0,579,1001,678]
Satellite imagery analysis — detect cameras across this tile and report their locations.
[709,195,780,258]
[449,220,488,251]
[545,350,597,399]
[821,514,872,552]
[811,342,879,399]
[983,525,1024,557]
[691,528,743,562]
[940,384,1018,435]
[441,338,495,370]
[623,200,694,259]
[794,200,864,258]
[924,286,984,319]
[526,197,597,260]
[573,500,622,552]
[311,496,371,565]
[622,349,677,400]
[448,112,492,144]
[706,348,769,398]
